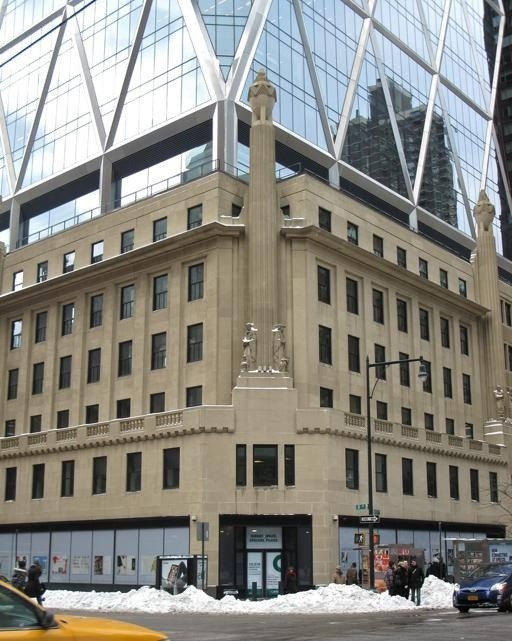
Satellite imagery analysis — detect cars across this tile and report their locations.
[0,575,171,641]
[453,561,512,612]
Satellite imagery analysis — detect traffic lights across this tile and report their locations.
[353,533,366,546]
[372,532,381,546]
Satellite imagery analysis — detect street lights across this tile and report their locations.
[366,354,428,593]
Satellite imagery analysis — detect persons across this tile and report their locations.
[12,557,45,609]
[272,321,286,365]
[331,562,357,586]
[493,385,512,418]
[384,560,425,607]
[285,566,298,594]
[241,322,257,370]
[426,552,448,579]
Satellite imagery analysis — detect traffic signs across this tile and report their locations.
[359,514,382,524]
